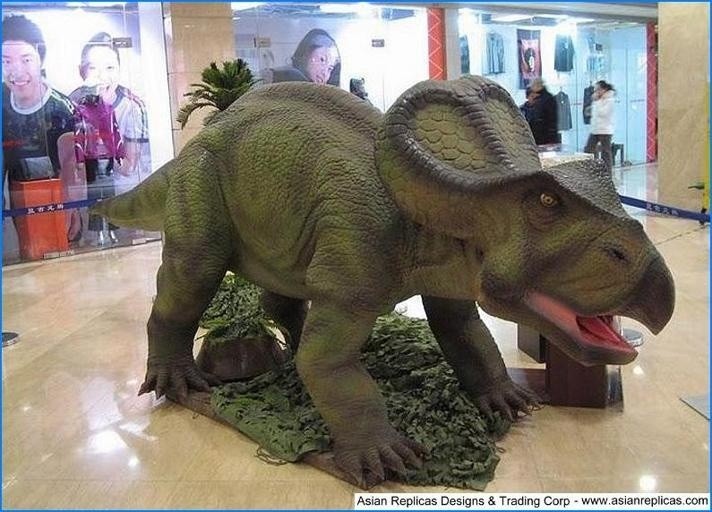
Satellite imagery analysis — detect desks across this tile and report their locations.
[9,178,70,261]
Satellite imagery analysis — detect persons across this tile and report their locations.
[1,12,87,248]
[522,46,538,80]
[64,32,151,183]
[291,26,341,89]
[583,79,617,176]
[524,76,560,143]
[520,84,536,112]
[73,90,128,247]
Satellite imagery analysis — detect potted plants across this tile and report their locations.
[175,275,286,386]
